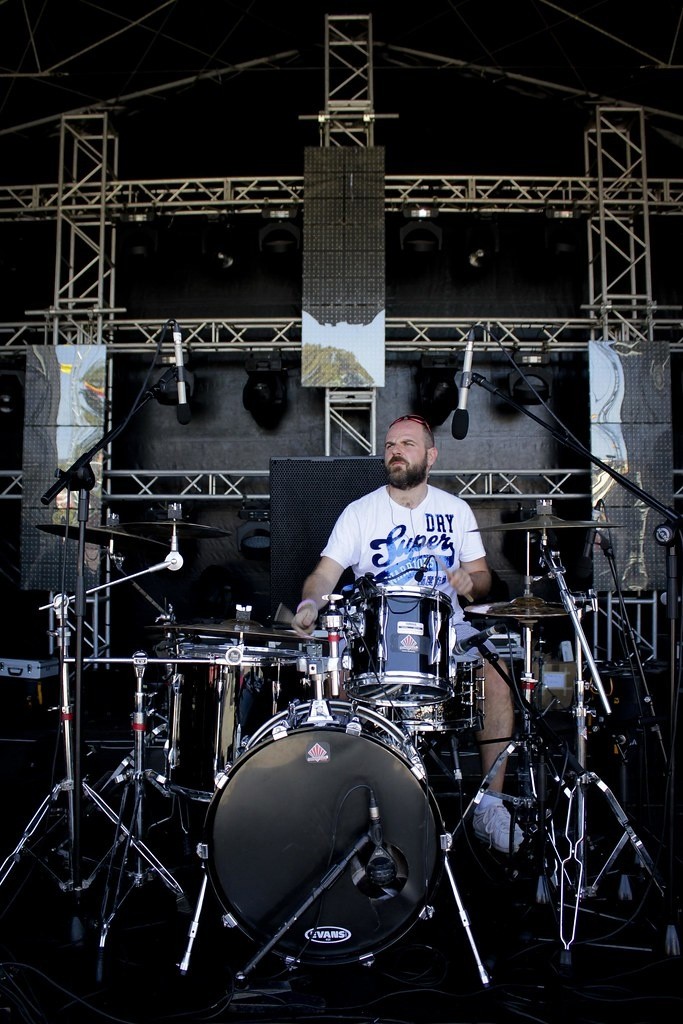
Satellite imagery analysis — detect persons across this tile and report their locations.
[292,414,524,856]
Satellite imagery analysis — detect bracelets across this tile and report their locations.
[296,599,318,613]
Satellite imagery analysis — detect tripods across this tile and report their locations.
[489,530,683,991]
[0,509,198,1007]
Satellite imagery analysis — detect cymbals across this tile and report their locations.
[465,514,630,533]
[98,519,232,541]
[461,594,579,619]
[34,522,172,553]
[144,617,329,644]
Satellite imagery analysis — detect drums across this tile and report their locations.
[340,584,456,708]
[201,698,452,970]
[400,654,484,733]
[161,641,311,805]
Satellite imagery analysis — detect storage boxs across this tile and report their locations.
[534,659,574,710]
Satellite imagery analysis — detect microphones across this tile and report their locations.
[173,323,191,424]
[582,502,602,557]
[415,555,432,582]
[452,326,477,441]
[453,623,505,656]
[367,789,398,886]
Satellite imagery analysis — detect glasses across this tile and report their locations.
[388,414,432,434]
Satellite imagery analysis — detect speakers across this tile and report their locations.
[268,455,389,627]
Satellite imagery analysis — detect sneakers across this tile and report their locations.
[472,804,524,853]
